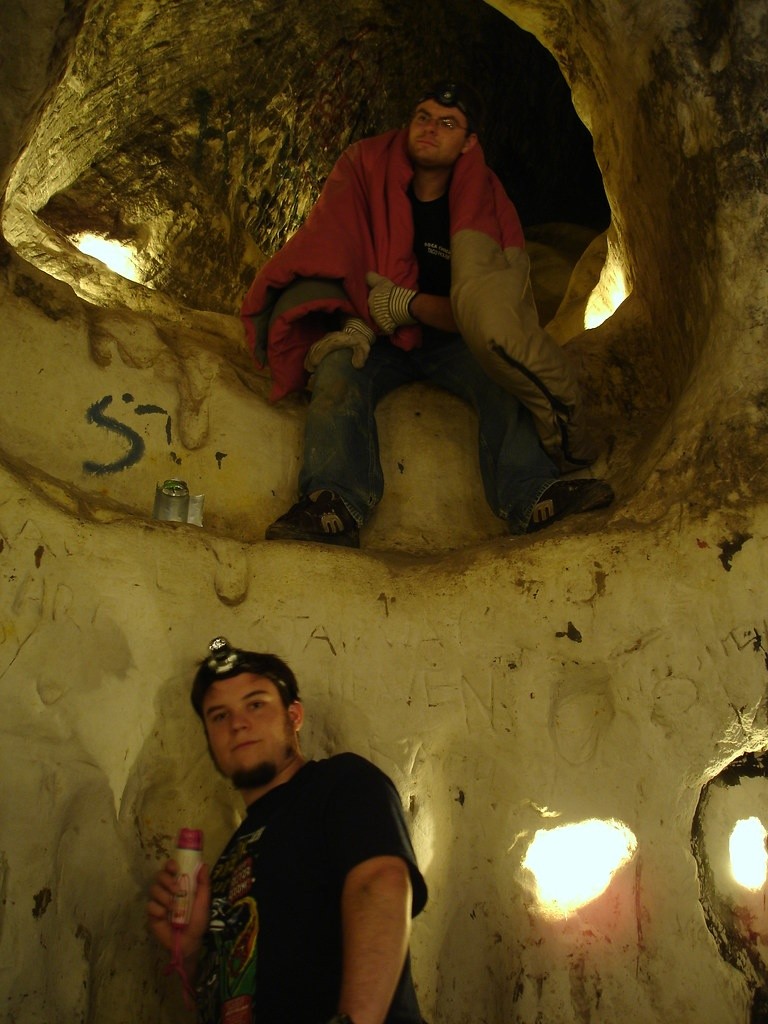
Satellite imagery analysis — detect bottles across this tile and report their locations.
[167,825,205,931]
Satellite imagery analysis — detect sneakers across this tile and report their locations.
[266,493,359,546]
[526,480,618,531]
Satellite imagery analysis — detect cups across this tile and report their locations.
[158,477,189,523]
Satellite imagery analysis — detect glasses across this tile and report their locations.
[413,112,470,131]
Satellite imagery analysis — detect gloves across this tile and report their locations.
[305,318,378,374]
[368,270,420,333]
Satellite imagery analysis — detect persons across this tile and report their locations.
[142,639,430,1022]
[230,74,620,532]
